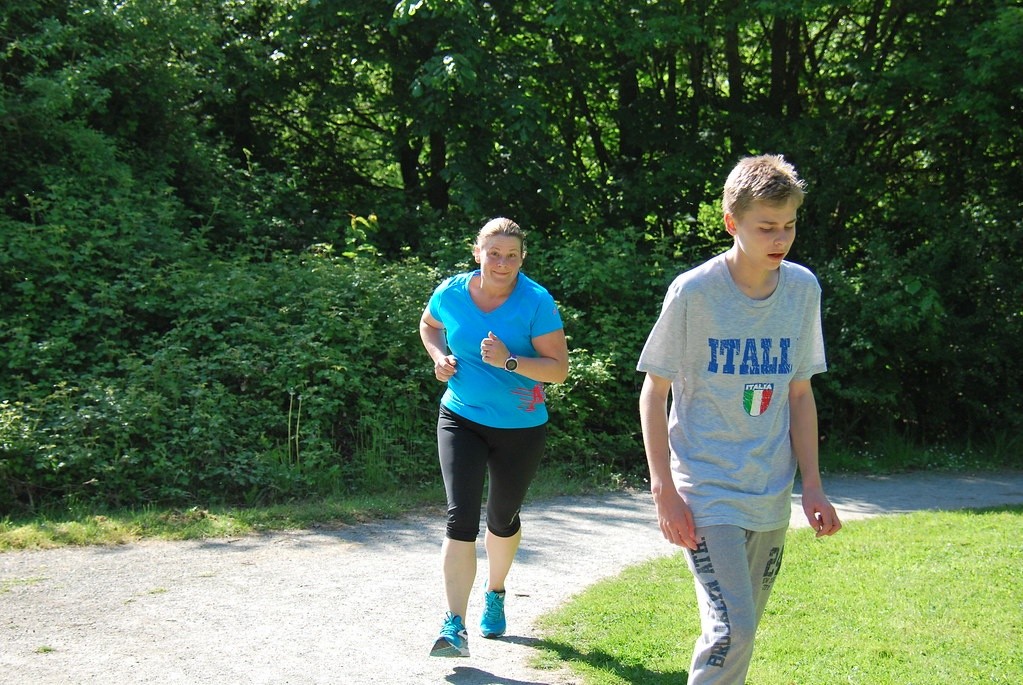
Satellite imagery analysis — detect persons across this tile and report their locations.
[418,217,569,660]
[636,152,843,685]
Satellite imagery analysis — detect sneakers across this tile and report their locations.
[430,614,470,658]
[480,578,507,638]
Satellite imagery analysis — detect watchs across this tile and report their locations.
[504,352,519,372]
